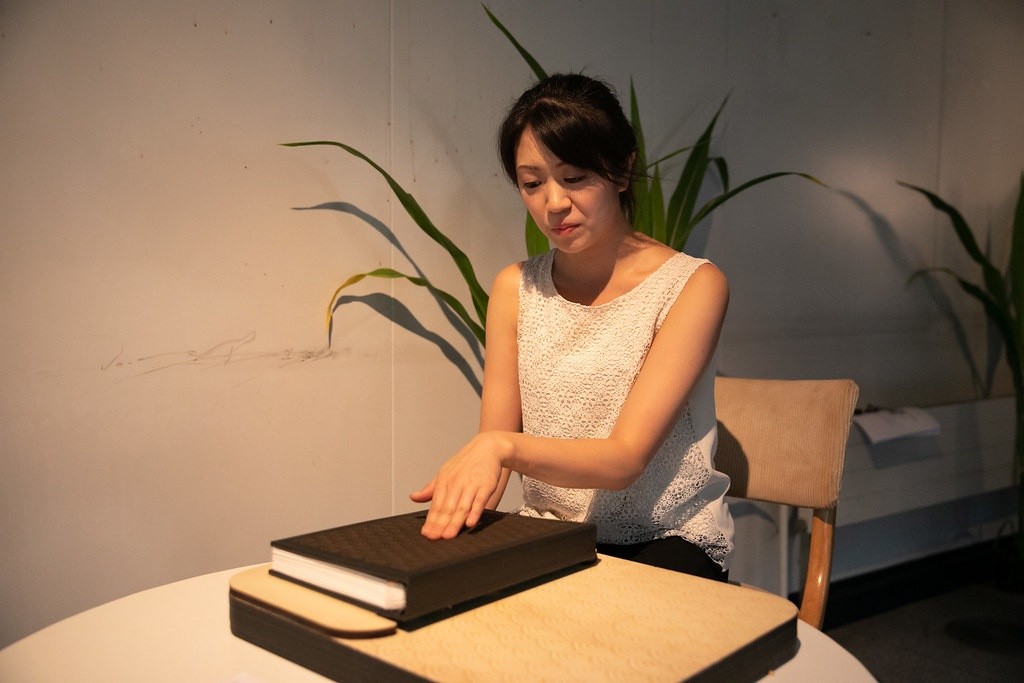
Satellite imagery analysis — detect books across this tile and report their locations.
[270,501,600,624]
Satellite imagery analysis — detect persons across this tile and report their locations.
[411,61,733,584]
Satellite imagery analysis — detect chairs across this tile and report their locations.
[713,376,859,631]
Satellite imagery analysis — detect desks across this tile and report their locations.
[0,553,881,683]
